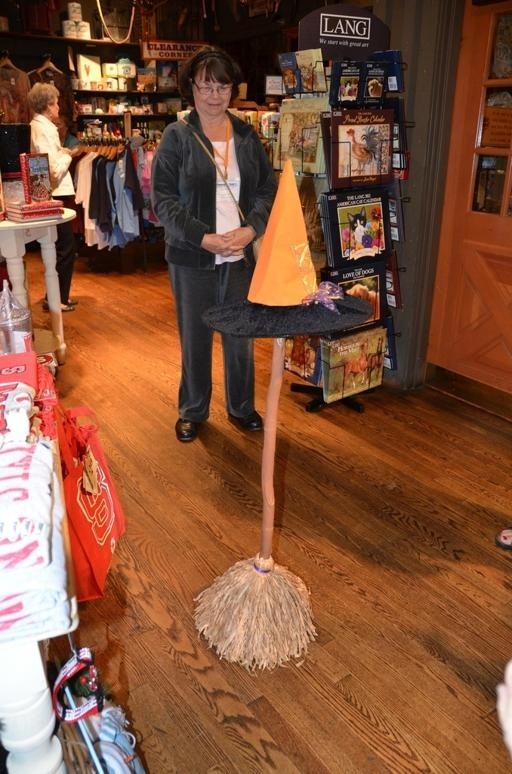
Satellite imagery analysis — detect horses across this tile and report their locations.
[332,335,383,390]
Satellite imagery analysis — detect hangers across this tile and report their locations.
[0,51,60,76]
[67,135,160,161]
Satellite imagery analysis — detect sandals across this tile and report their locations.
[42,296,79,312]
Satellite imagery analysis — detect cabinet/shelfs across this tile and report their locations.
[0,0,194,136]
[422,1,511,399]
[264,50,416,413]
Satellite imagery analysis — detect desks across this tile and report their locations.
[0,205,77,367]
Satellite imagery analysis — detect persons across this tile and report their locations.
[151,46,277,441]
[495,527,512,550]
[27,82,78,312]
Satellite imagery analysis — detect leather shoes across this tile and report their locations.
[174,416,202,442]
[228,408,263,432]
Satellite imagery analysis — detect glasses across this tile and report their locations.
[193,81,233,96]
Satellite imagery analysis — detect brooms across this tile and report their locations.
[193,338,318,672]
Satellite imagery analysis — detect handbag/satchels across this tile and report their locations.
[252,233,265,263]
[52,405,127,603]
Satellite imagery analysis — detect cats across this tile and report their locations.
[347,207,369,242]
